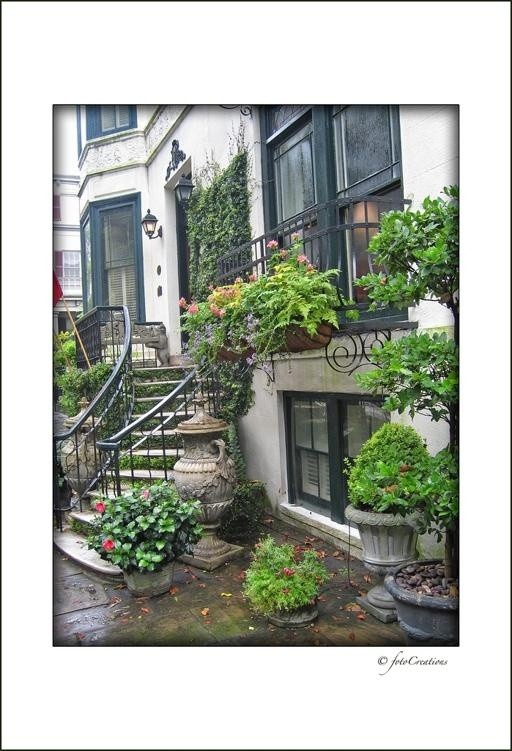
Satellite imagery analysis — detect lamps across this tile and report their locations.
[141,208,162,239]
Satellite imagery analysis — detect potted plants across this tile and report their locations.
[244,532,330,629]
[342,423,434,610]
[382,187,458,643]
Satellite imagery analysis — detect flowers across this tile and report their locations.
[178,231,361,395]
[76,478,206,574]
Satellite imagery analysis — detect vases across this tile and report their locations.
[121,560,174,599]
